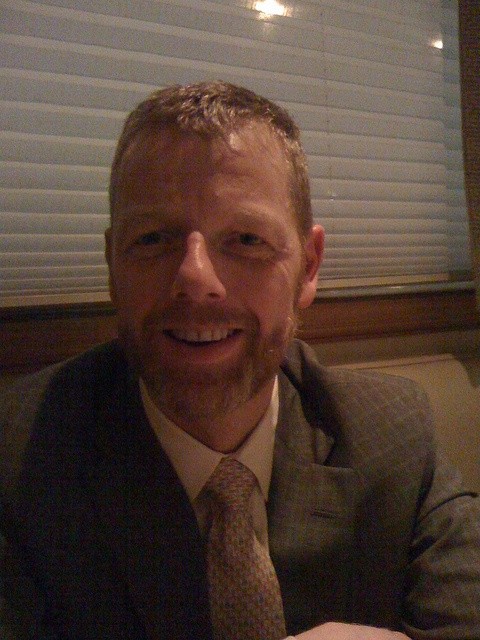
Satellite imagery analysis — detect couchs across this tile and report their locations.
[323,349,480,494]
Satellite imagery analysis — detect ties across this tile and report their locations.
[204,456,285,636]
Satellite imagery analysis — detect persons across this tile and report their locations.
[0,82,480,640]
